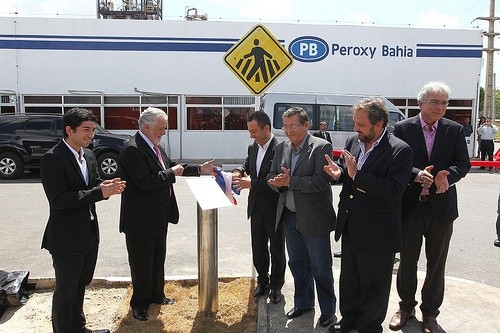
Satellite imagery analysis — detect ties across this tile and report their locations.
[154,144,166,172]
[323,132,325,137]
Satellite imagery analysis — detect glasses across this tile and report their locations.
[425,100,449,108]
[282,123,304,131]
[321,125,327,127]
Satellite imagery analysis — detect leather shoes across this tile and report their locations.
[270,288,281,303]
[389,306,415,331]
[158,297,176,305]
[287,307,313,319]
[421,311,437,333]
[254,286,266,297]
[80,327,110,333]
[329,318,357,333]
[320,313,335,327]
[358,327,383,333]
[133,308,148,321]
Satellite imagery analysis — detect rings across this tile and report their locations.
[440,184,444,187]
[348,157,352,160]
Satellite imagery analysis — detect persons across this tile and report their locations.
[229,110,287,303]
[478,117,498,171]
[462,116,487,158]
[268,105,337,328]
[40,107,126,333]
[119,107,216,321]
[312,122,333,145]
[389,81,472,333]
[324,97,413,333]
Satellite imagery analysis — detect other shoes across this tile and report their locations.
[477,167,485,170]
[493,239,500,246]
[476,157,480,158]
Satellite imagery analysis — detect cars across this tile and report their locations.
[0,111,139,181]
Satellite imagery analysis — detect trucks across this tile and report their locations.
[260,91,410,185]
